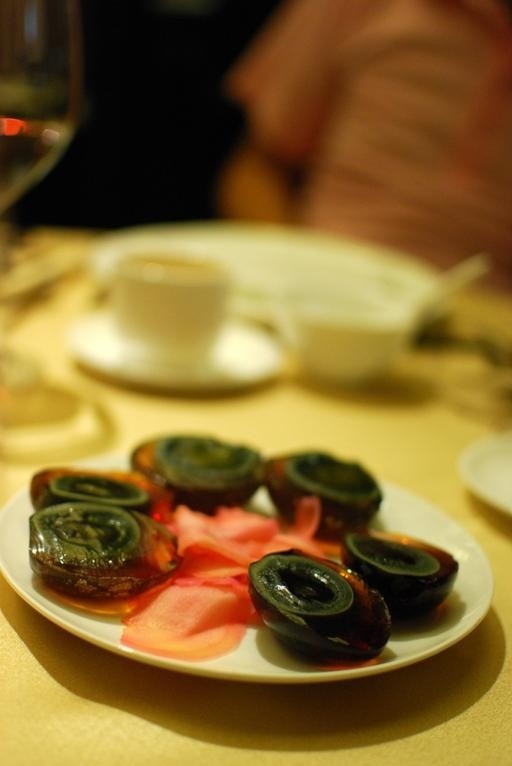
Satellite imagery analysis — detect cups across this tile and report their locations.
[292,266,425,391]
[109,254,236,354]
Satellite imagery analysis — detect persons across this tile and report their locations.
[212,0,511,293]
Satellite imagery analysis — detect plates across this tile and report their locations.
[65,306,283,399]
[1,444,496,687]
[462,437,510,521]
